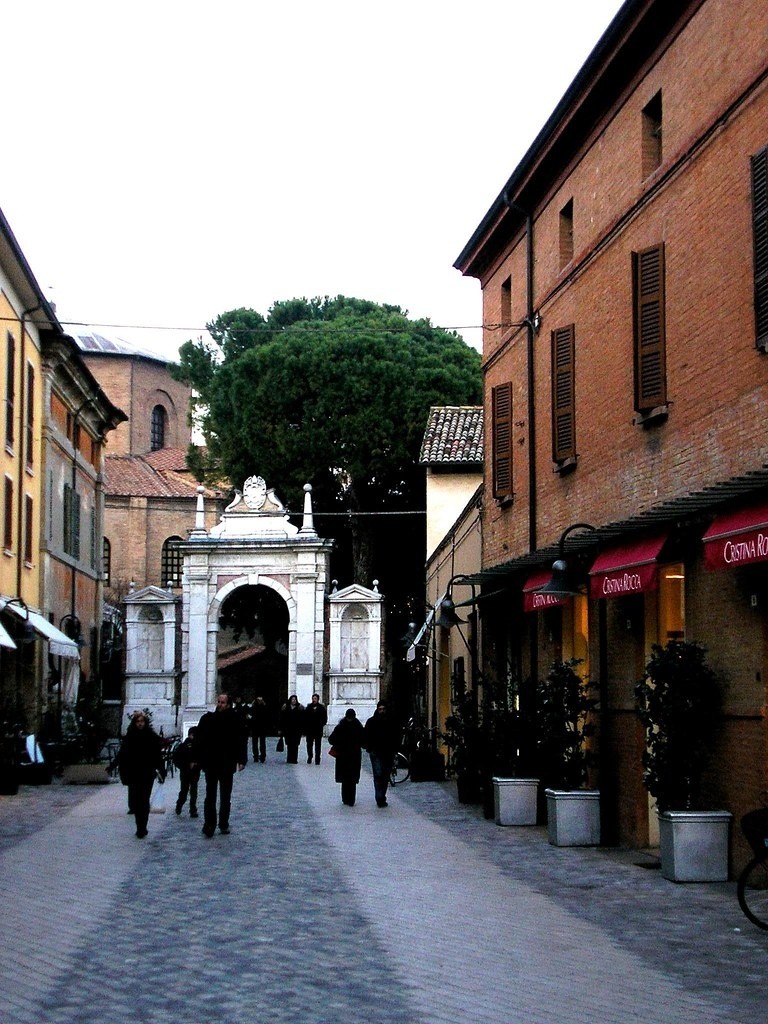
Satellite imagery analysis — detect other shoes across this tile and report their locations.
[203,826,214,837]
[137,829,148,838]
[176,804,182,815]
[128,808,135,814]
[190,809,199,817]
[221,827,230,833]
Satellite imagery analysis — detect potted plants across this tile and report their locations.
[405,695,448,782]
[436,646,604,848]
[634,630,733,884]
[0,667,115,795]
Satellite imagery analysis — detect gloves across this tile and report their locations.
[105,762,116,772]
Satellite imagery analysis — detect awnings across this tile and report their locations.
[407,594,449,662]
[589,531,668,599]
[0,623,16,648]
[7,604,80,660]
[522,571,571,611]
[701,497,768,571]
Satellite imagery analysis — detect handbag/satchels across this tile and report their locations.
[155,766,165,783]
[328,744,341,757]
[276,736,284,752]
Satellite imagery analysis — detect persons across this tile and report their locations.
[193,693,267,837]
[278,695,327,765]
[328,703,400,807]
[105,712,166,838]
[174,727,201,817]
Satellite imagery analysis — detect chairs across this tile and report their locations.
[108,742,121,779]
[160,735,183,779]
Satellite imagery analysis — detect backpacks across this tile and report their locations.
[173,744,191,770]
